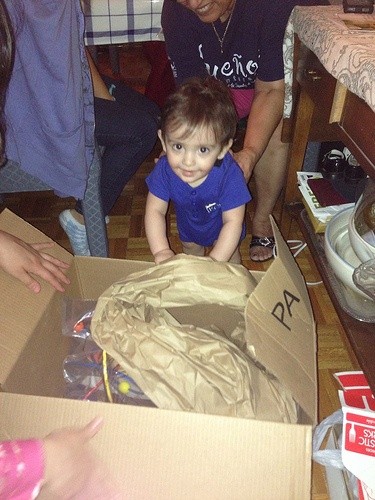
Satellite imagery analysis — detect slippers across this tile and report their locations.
[249,234,275,262]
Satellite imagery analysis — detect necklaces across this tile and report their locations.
[211,7,235,55]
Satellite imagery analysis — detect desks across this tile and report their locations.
[281,7,375,399]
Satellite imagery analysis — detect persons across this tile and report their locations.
[144,74,250,265]
[0,227,71,294]
[0,416,111,500]
[161,0,292,261]
[5,0,162,257]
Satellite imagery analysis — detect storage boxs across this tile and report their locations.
[302,196,326,234]
[0,206,319,500]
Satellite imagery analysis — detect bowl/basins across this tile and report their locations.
[348,212,375,263]
[324,206,375,303]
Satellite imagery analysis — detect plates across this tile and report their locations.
[353,259,375,300]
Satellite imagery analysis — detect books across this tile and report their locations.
[338,12,375,33]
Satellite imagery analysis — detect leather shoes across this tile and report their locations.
[320,148,346,181]
[346,154,367,184]
